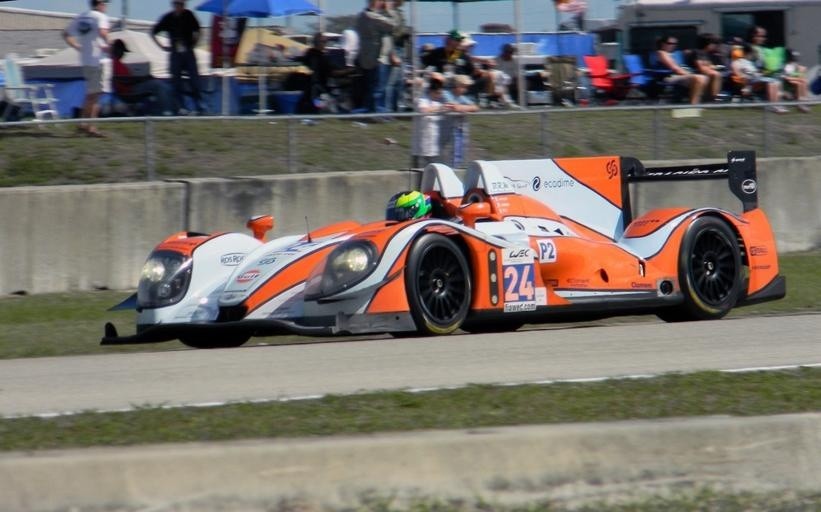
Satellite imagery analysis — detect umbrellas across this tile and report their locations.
[196,1,327,116]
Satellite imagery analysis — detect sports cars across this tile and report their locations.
[103,147,788,348]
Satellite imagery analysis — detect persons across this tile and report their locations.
[63,0,118,140]
[300,1,525,118]
[109,39,202,117]
[408,77,449,169]
[648,24,815,116]
[149,1,209,117]
[440,73,483,168]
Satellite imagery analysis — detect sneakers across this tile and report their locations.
[767,105,789,114]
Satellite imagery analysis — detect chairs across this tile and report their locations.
[583,53,635,105]
[620,43,732,104]
[0,55,60,123]
[107,72,159,115]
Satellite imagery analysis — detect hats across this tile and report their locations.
[501,43,518,53]
[445,29,479,51]
[114,39,130,52]
[451,74,476,88]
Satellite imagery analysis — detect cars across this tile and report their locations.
[321,31,344,49]
